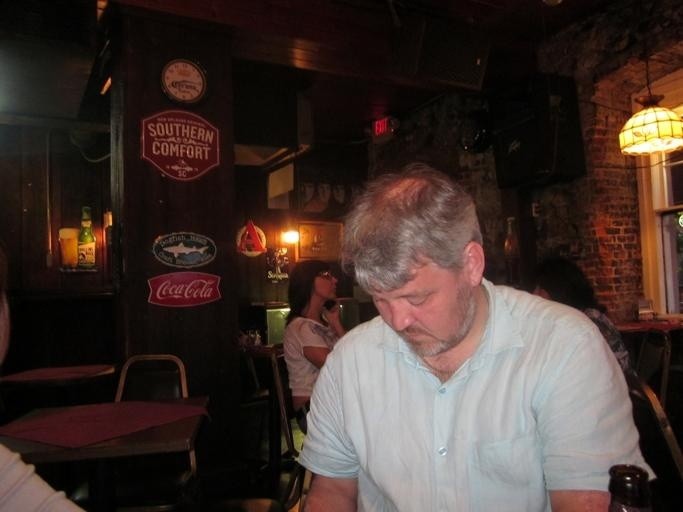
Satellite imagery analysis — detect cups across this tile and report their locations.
[59,227,77,273]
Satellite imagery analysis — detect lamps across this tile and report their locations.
[619,56,683,156]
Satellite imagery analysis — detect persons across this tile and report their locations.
[292,162,662,511]
[527,259,634,378]
[0,233,88,512]
[279,258,350,435]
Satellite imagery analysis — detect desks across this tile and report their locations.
[0,395,211,512]
[615,320,683,364]
[0,364,115,419]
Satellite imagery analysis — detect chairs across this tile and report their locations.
[635,328,672,410]
[624,367,683,512]
[116,354,198,512]
[270,344,305,512]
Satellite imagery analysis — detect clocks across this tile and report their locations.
[159,59,207,107]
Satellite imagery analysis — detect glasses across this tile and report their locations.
[319,271,337,279]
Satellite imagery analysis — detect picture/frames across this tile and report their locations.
[294,220,345,263]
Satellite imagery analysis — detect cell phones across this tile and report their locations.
[325,301,338,309]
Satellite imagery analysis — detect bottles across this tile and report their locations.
[603,464,654,512]
[78,204,96,270]
[504,217,518,255]
